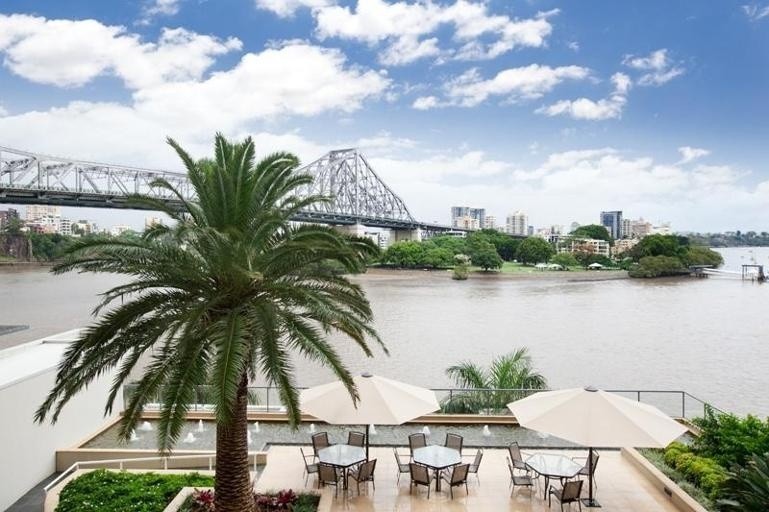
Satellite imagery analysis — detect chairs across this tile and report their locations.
[300,431,599,512]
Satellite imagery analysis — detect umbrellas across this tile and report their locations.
[503,386,690,502]
[292,370,441,464]
